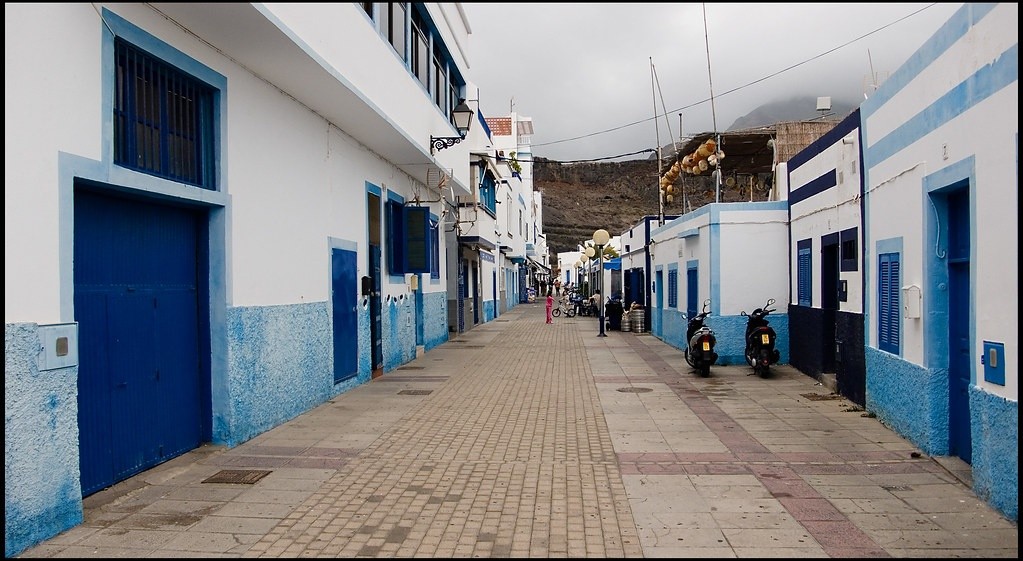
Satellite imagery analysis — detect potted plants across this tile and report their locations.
[497,151,522,176]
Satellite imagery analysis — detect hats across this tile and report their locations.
[596,290,600,294]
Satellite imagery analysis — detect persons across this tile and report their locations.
[545,290,555,324]
[559,290,600,318]
[539,277,578,297]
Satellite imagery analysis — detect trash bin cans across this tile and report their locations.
[606,301,623,331]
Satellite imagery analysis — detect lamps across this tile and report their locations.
[430,98,475,156]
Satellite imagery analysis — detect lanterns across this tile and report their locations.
[660,138,725,202]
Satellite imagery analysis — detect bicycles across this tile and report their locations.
[551,300,576,318]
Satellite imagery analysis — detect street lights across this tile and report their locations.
[574,261,581,289]
[585,246,595,297]
[592,229,609,336]
[580,254,587,296]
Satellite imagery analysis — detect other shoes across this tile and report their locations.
[550,321,554,323]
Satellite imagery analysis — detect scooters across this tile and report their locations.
[681,299,719,379]
[740,298,780,378]
